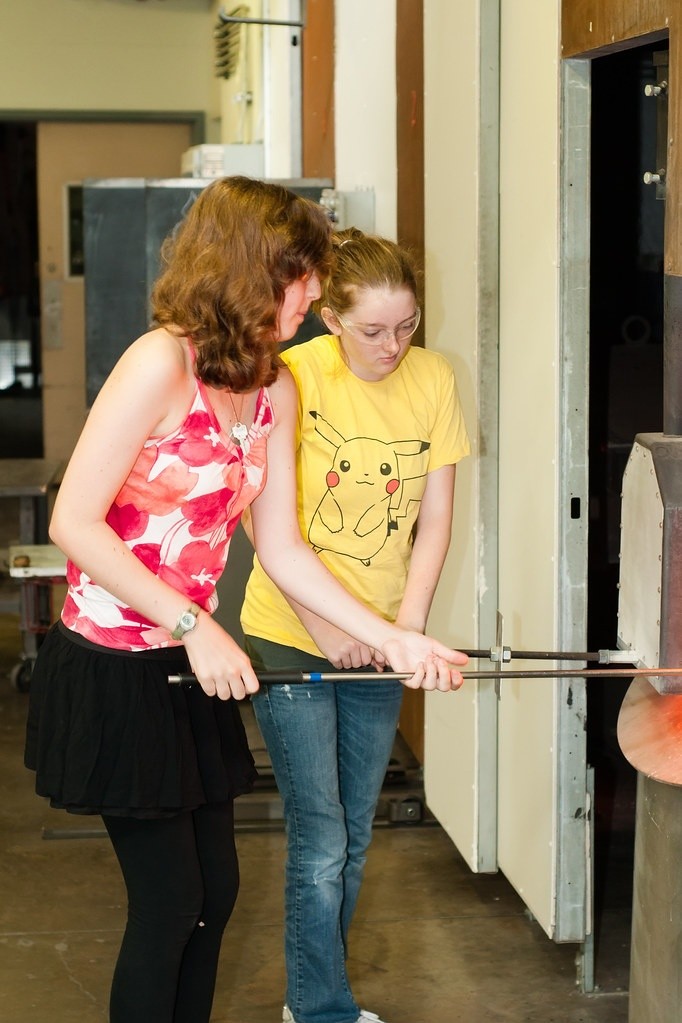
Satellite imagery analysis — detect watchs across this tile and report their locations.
[170,602,202,641]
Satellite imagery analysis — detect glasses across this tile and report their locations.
[326,305,421,346]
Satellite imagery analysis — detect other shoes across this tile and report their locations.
[282,1003,385,1023]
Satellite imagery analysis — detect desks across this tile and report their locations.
[0,457,71,688]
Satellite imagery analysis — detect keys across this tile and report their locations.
[233,422,248,456]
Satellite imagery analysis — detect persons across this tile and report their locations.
[24,175,467,1023]
[240,226,472,1023]
